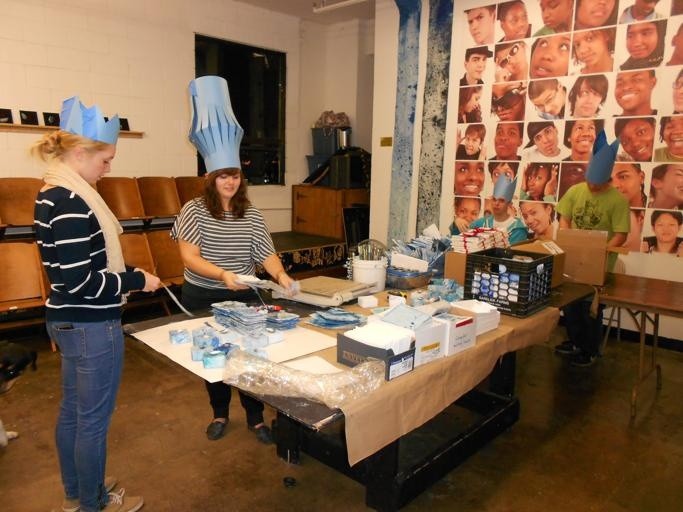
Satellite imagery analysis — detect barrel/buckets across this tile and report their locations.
[351,255,389,295]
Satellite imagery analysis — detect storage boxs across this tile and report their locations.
[557,229,608,285]
[444,238,565,293]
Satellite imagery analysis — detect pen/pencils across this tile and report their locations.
[358,243,383,261]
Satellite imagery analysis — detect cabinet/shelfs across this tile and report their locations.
[290,184,369,240]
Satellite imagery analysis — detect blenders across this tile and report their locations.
[330,126,363,189]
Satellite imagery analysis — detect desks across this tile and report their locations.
[118,278,605,510]
[591,273,683,422]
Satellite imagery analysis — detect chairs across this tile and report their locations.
[0,177,206,354]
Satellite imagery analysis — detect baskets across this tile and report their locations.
[463,248,554,319]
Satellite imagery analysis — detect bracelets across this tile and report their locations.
[276,271,286,283]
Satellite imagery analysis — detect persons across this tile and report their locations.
[451,1,683,258]
[28,127,159,512]
[168,168,300,445]
[552,180,630,359]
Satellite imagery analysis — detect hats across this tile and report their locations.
[493,174,517,201]
[585,129,621,184]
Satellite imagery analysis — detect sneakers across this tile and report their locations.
[206,416,227,440]
[248,422,271,443]
[61,475,144,512]
[555,340,599,367]
